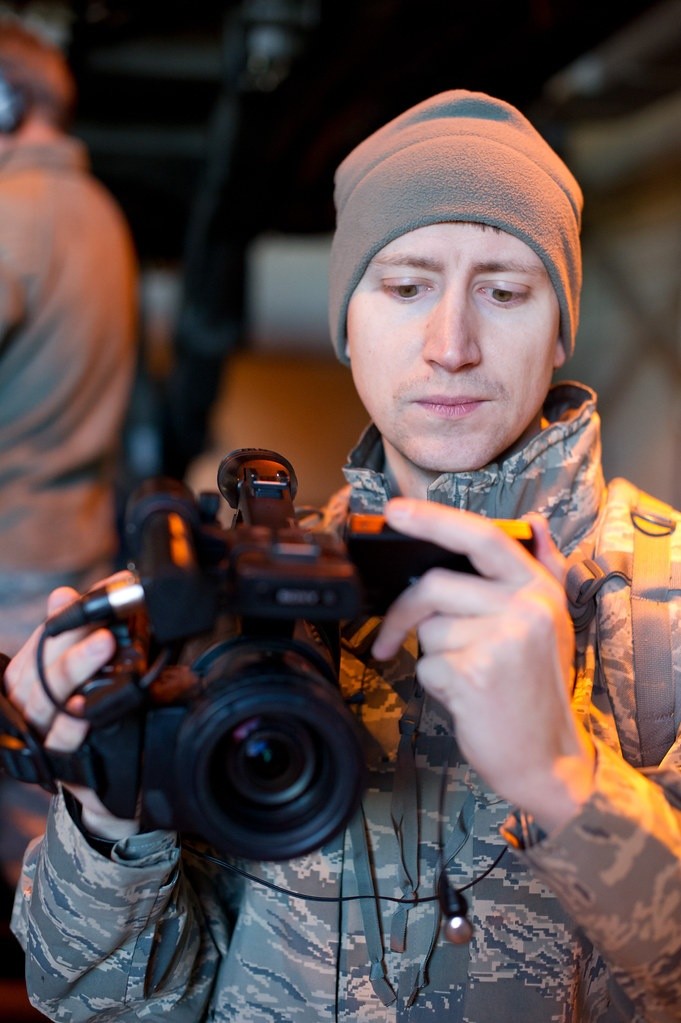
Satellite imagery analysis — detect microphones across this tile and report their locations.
[123,476,218,641]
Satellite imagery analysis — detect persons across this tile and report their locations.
[0,16,139,678]
[7,89,681,1023]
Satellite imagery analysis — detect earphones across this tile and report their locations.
[436,877,473,946]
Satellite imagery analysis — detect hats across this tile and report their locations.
[328,87,583,365]
